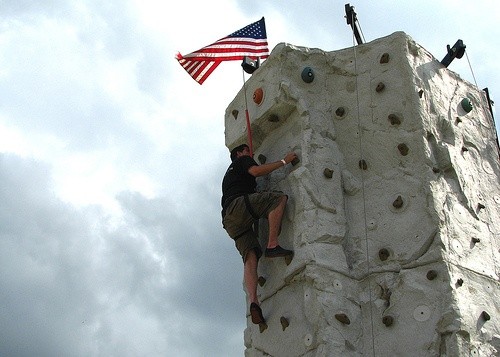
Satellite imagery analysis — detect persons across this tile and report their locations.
[221,144,297,324]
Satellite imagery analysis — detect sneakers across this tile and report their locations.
[250,302,264,325]
[265,245,293,257]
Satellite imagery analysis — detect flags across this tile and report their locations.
[175,16,270,86]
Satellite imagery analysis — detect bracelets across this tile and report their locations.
[280,159,288,165]
